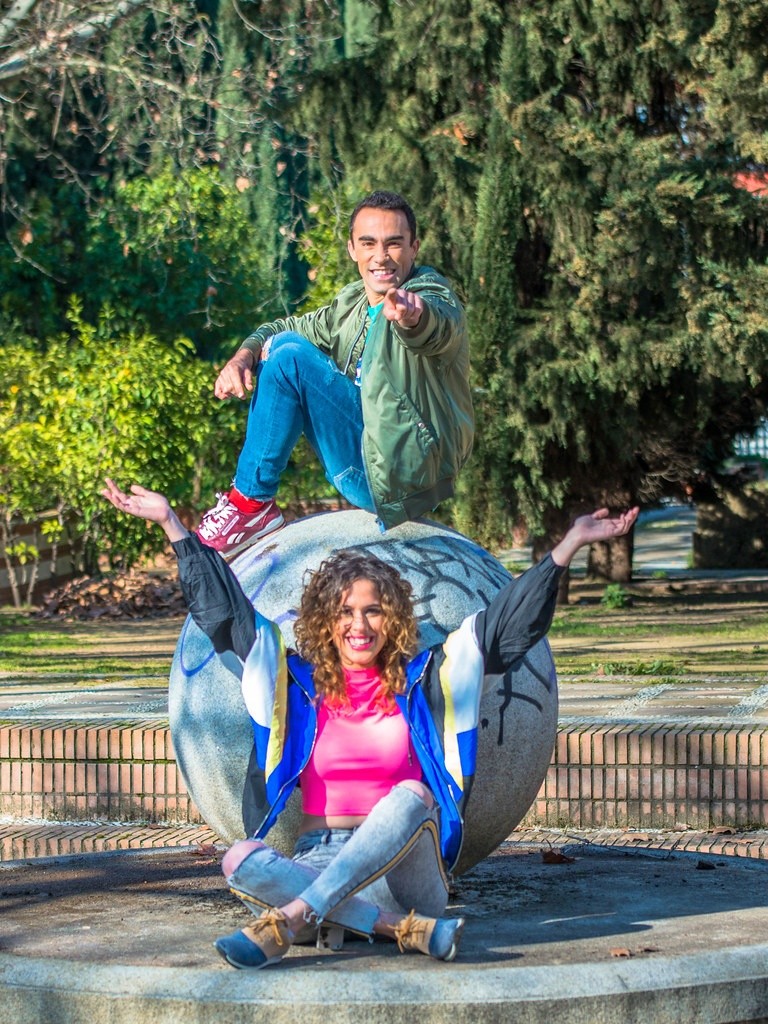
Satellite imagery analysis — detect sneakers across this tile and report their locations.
[213,906,295,971]
[195,491,284,561]
[386,908,465,961]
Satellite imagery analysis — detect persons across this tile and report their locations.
[102,477,640,972]
[194,189,477,566]
